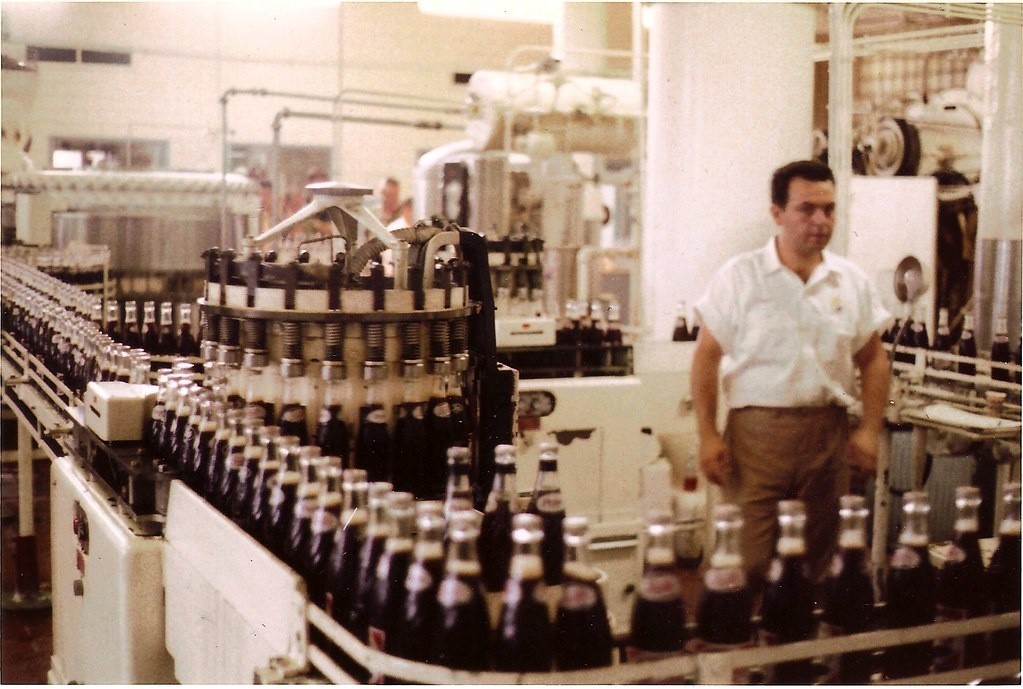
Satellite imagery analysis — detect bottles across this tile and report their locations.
[0,240,1022,683]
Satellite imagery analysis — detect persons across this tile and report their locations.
[689,159,893,599]
[374,173,416,229]
[257,166,331,240]
